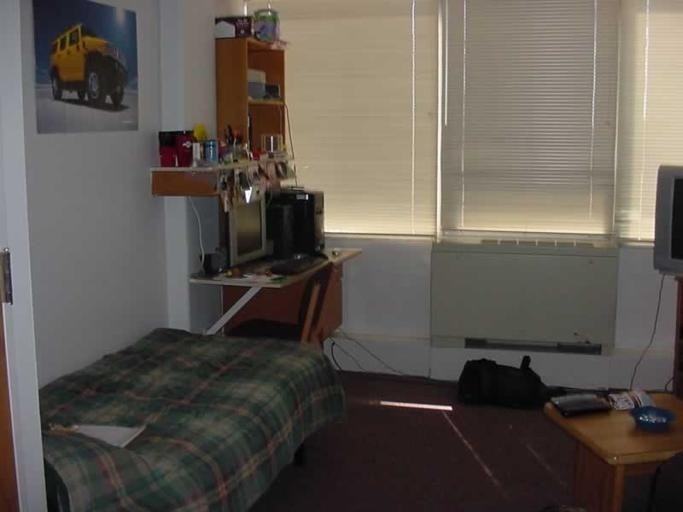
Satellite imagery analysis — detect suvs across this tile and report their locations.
[48,24,127,105]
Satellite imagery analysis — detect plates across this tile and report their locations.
[632,407,675,430]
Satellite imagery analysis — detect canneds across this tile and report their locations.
[203,140,219,166]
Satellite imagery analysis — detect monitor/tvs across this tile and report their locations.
[227,184,266,272]
[654,166,683,277]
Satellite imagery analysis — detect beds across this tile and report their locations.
[38,327,346,512]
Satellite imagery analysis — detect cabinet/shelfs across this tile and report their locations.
[216,37,286,155]
[146,157,297,199]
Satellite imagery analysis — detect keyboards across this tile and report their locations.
[270,255,325,276]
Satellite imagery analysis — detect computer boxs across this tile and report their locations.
[265,189,324,255]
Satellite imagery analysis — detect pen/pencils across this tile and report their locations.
[223,125,241,145]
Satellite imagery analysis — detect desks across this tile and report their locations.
[544,392,682,512]
[188,245,362,349]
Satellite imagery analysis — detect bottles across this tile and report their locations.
[194,122,219,166]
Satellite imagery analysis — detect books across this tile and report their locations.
[72,424,146,449]
[210,268,285,286]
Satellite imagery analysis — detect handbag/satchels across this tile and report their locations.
[456,354,552,411]
